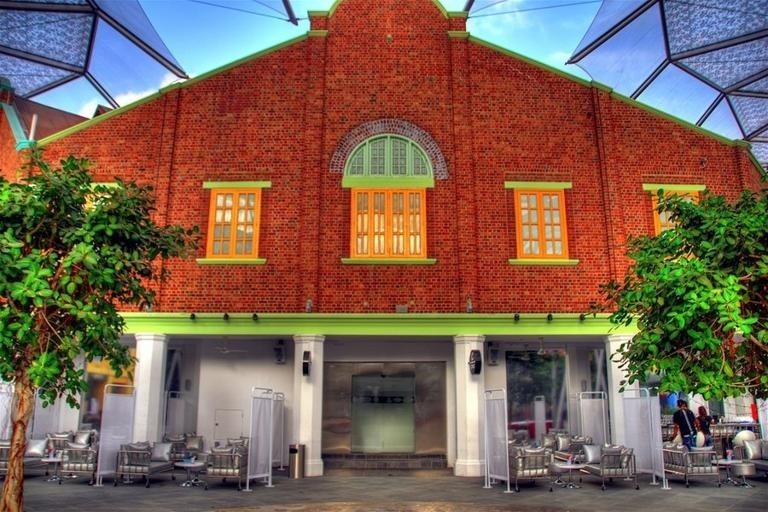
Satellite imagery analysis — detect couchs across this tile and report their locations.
[508,429,768,491]
[0,429,247,494]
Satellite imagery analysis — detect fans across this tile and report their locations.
[507,336,563,361]
[211,337,247,354]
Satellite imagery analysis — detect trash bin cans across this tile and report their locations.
[289,444,304,480]
